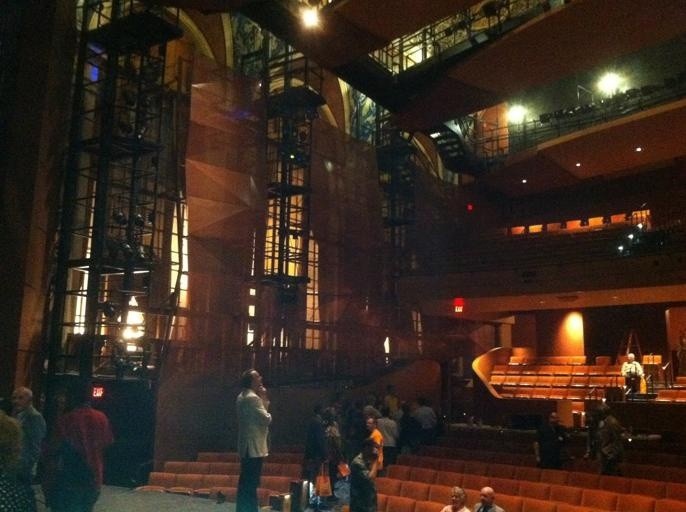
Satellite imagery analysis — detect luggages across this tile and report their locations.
[289,479,314,512]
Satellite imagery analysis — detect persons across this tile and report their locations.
[536,412,571,468]
[503,414,534,467]
[582,403,626,475]
[0,382,114,512]
[445,349,464,422]
[475,486,505,512]
[261,385,438,512]
[234,369,273,512]
[621,353,646,398]
[456,415,482,448]
[441,486,471,512]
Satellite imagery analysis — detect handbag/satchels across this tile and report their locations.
[337,464,351,478]
[315,463,332,498]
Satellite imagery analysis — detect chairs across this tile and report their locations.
[488,352,685,406]
[492,208,653,236]
[130,444,685,512]
